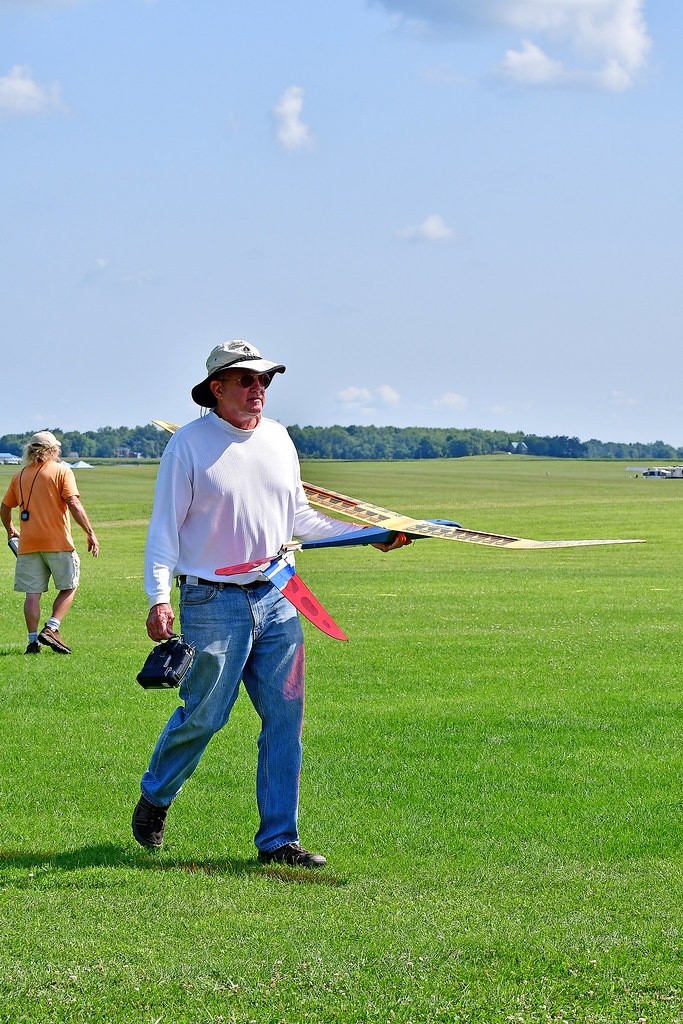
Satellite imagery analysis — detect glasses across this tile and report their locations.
[218,373,271,389]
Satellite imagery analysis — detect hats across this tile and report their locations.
[191,338,286,408]
[30,431,62,449]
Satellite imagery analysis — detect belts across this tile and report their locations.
[179,574,271,590]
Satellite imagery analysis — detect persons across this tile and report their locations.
[131,342,412,870]
[0,431,100,654]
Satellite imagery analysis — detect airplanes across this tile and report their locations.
[150,419,650,643]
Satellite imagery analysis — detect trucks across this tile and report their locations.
[649,466,683,478]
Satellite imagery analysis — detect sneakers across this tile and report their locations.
[258,842,327,867]
[24,641,41,655]
[37,623,72,654]
[132,794,171,849]
